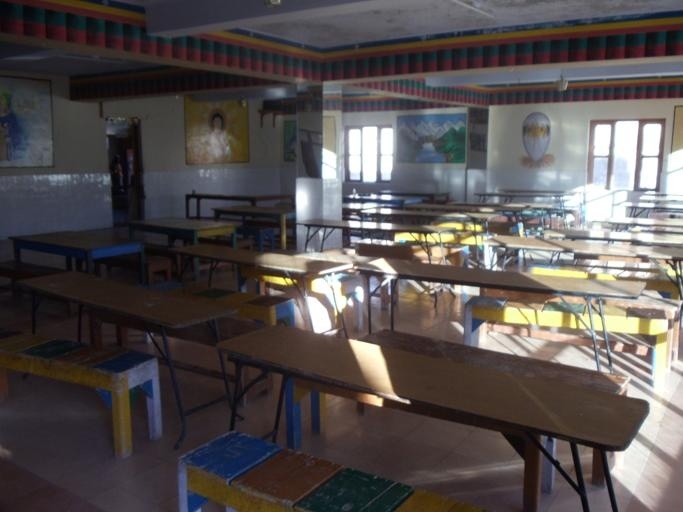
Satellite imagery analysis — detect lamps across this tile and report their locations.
[553,67,568,92]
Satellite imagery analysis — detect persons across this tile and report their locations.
[201,109,231,164]
[1,92,21,159]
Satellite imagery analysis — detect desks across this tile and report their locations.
[4,192,682,374]
[208,321,650,512]
[25,270,243,447]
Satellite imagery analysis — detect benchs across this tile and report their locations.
[1,326,165,460]
[352,327,635,494]
[176,429,489,512]
